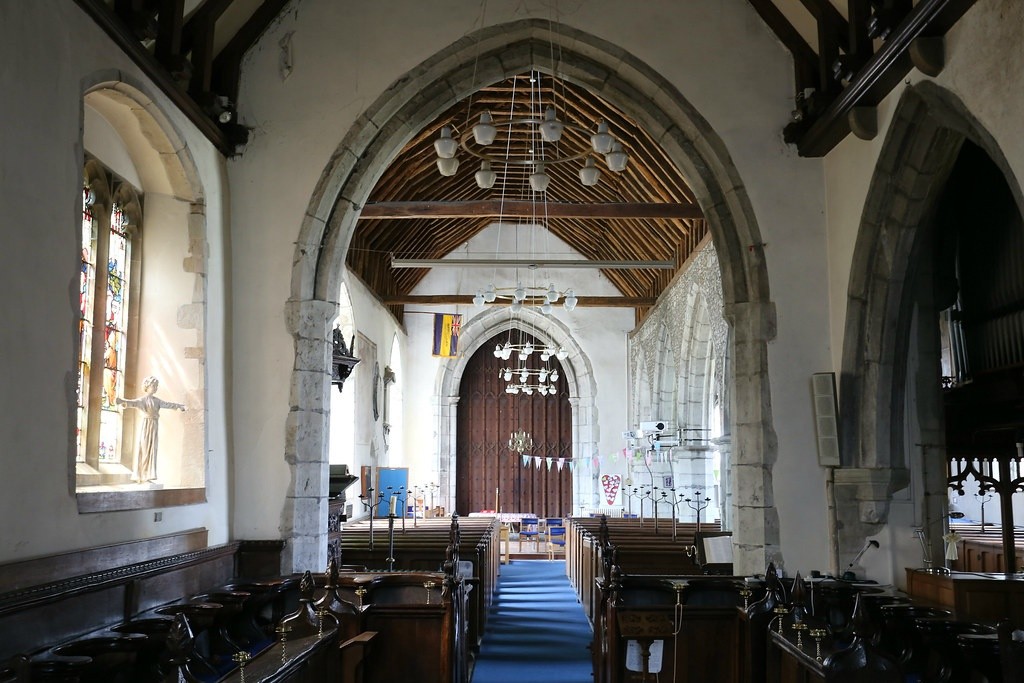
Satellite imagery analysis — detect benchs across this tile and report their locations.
[564,516,733,635]
[342,516,500,650]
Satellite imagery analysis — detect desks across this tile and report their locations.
[469,512,537,533]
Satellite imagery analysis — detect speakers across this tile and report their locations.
[813,373,842,467]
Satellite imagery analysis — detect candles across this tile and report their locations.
[389,495,397,514]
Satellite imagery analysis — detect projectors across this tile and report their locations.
[640,421,669,432]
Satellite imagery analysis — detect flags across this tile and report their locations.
[431,313,462,359]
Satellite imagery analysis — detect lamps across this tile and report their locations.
[436,0,630,396]
[840,539,880,582]
[916,511,965,573]
[211,102,232,123]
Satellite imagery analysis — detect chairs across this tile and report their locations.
[519,518,565,561]
